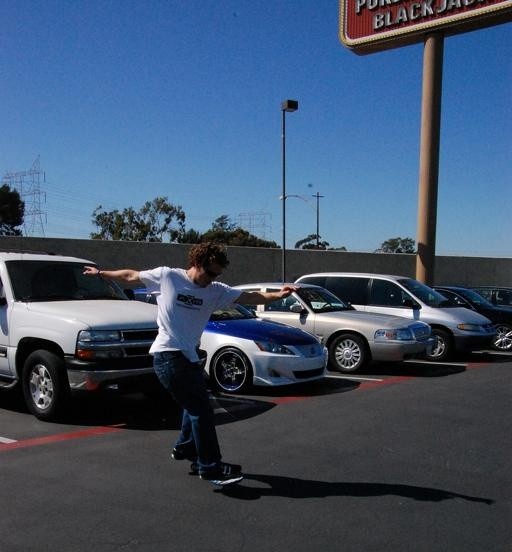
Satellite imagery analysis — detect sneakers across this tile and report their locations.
[170,445,200,463]
[198,461,243,482]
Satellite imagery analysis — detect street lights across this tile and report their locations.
[280,99,299,285]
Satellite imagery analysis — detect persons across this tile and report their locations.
[82,241,302,480]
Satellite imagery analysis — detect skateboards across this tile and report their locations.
[185,453,244,488]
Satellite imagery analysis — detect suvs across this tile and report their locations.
[0,250,176,420]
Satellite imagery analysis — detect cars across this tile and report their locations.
[466,286,510,310]
[132,282,328,393]
[432,285,512,352]
[295,273,497,361]
[229,284,432,374]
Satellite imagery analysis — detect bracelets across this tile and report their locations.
[97,269,101,280]
